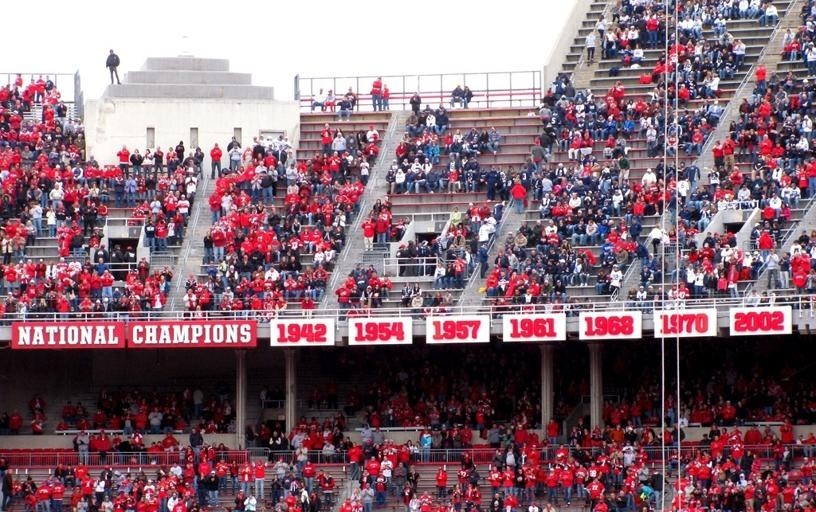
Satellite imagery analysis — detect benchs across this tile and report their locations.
[486,0,816,305]
[2,104,542,311]
[3,392,815,509]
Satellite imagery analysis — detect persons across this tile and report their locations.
[534,339,815,510]
[106,49,122,84]
[1,340,533,511]
[1,71,532,316]
[535,2,814,309]
